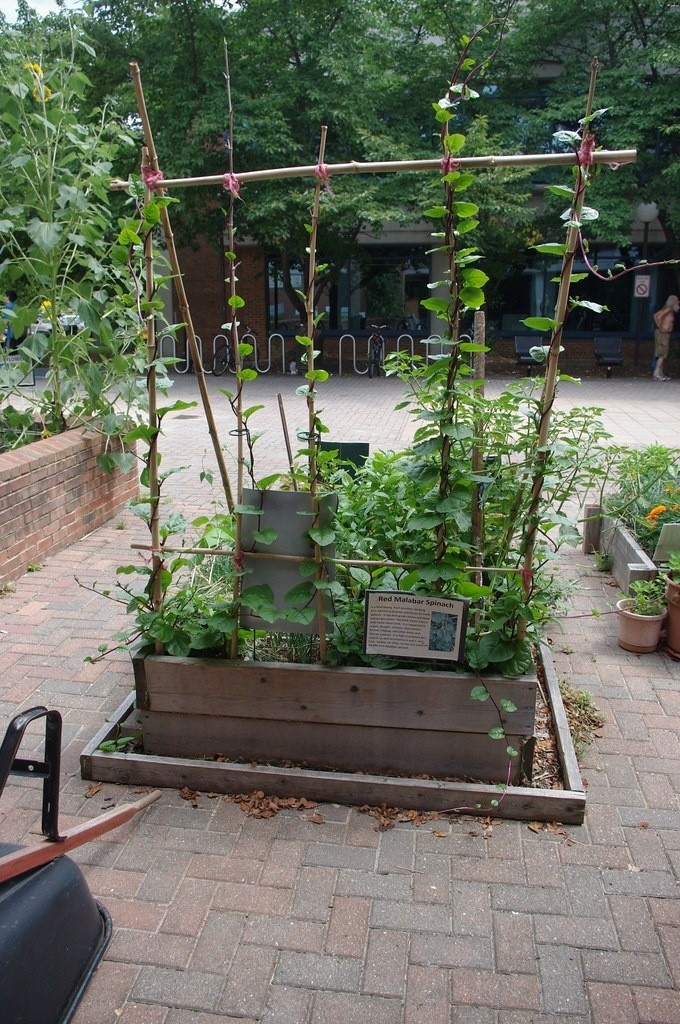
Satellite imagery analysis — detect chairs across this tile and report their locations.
[594,336,624,378]
[515,336,544,377]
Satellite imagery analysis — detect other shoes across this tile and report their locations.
[663,376,671,381]
[652,375,666,381]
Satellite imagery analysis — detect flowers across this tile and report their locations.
[23,62,43,83]
[647,489,680,529]
[33,85,51,102]
[41,301,52,320]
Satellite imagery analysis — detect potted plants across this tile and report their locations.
[616,571,668,654]
[665,550,680,659]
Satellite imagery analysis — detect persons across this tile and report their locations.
[0,290,18,356]
[653,296,680,381]
